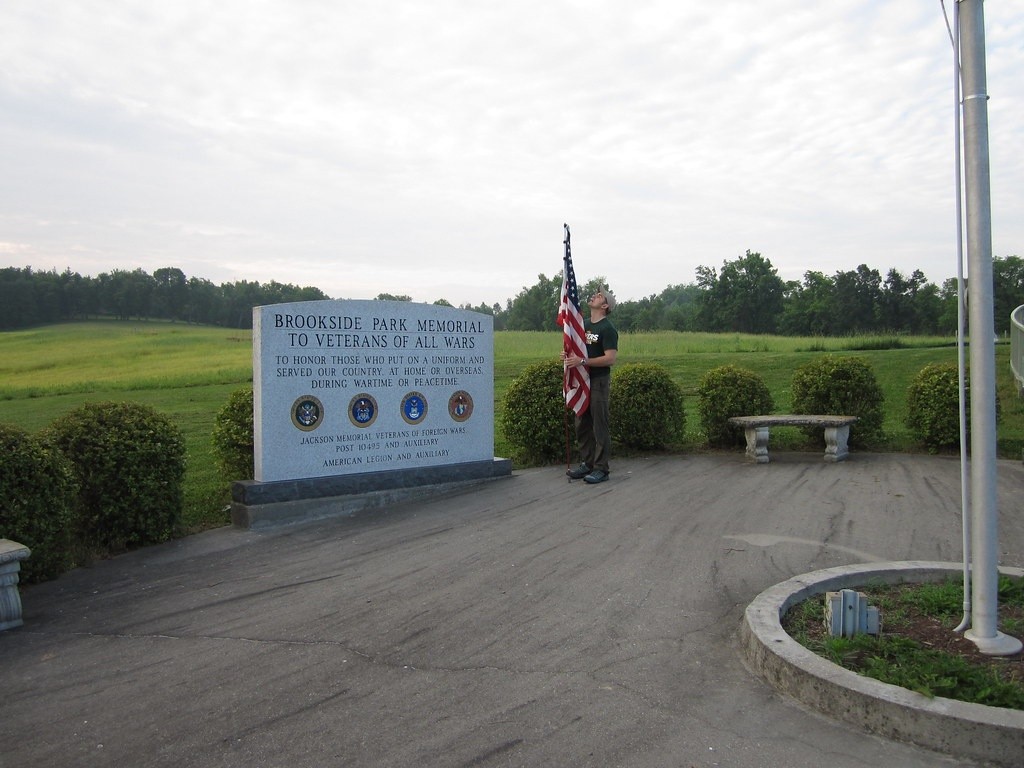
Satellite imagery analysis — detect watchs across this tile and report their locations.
[580,358,586,365]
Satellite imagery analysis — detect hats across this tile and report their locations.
[597,283,617,312]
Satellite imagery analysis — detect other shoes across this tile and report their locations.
[583,470,610,483]
[566,462,596,479]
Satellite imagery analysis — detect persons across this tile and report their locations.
[559,283,619,484]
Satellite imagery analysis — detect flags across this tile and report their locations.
[554,223,590,416]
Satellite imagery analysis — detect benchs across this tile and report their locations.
[729,414,860,464]
[0,538,32,631]
